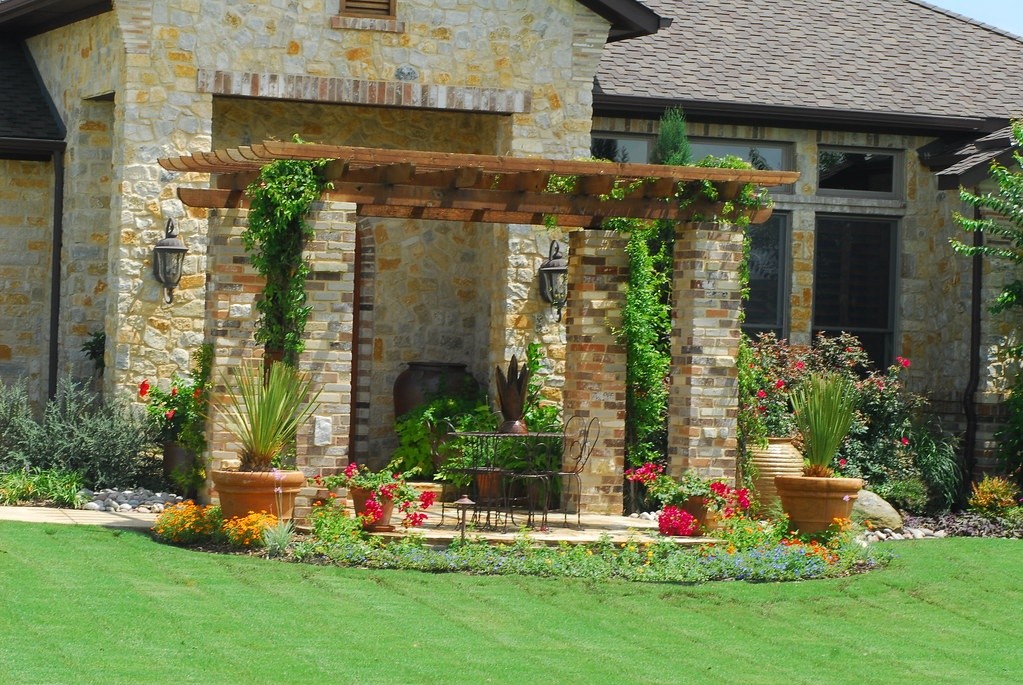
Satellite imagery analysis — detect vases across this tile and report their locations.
[162,440,193,485]
[678,495,709,537]
[346,483,398,528]
[742,435,806,520]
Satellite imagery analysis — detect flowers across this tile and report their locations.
[743,356,792,439]
[306,458,437,530]
[139,343,212,487]
[624,464,750,537]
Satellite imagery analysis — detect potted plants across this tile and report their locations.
[510,411,568,510]
[773,372,864,545]
[434,427,527,506]
[193,361,326,527]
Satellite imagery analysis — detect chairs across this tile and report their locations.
[437,411,602,530]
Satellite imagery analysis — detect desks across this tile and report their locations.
[447,430,576,530]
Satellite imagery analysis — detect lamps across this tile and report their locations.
[538,240,568,323]
[153,218,188,304]
